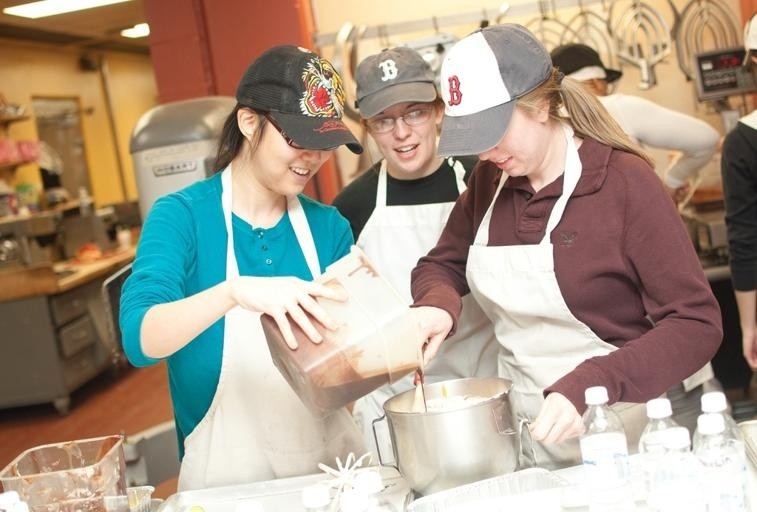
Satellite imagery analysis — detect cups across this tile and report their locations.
[114,225,131,251]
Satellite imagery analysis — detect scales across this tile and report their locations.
[694,48,757,130]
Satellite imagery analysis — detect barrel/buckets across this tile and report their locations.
[262,244,421,414]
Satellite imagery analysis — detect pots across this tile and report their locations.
[374,373,522,493]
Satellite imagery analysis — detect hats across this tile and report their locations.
[235,44,365,153]
[550,44,622,82]
[743,14,757,66]
[435,23,553,159]
[353,45,437,119]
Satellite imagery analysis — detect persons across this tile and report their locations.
[117,42,376,496]
[403,23,724,462]
[717,10,756,376]
[332,43,516,469]
[548,42,723,213]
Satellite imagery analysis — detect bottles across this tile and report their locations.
[576,385,749,512]
[1,181,90,219]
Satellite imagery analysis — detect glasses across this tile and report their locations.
[263,113,340,154]
[364,101,439,134]
